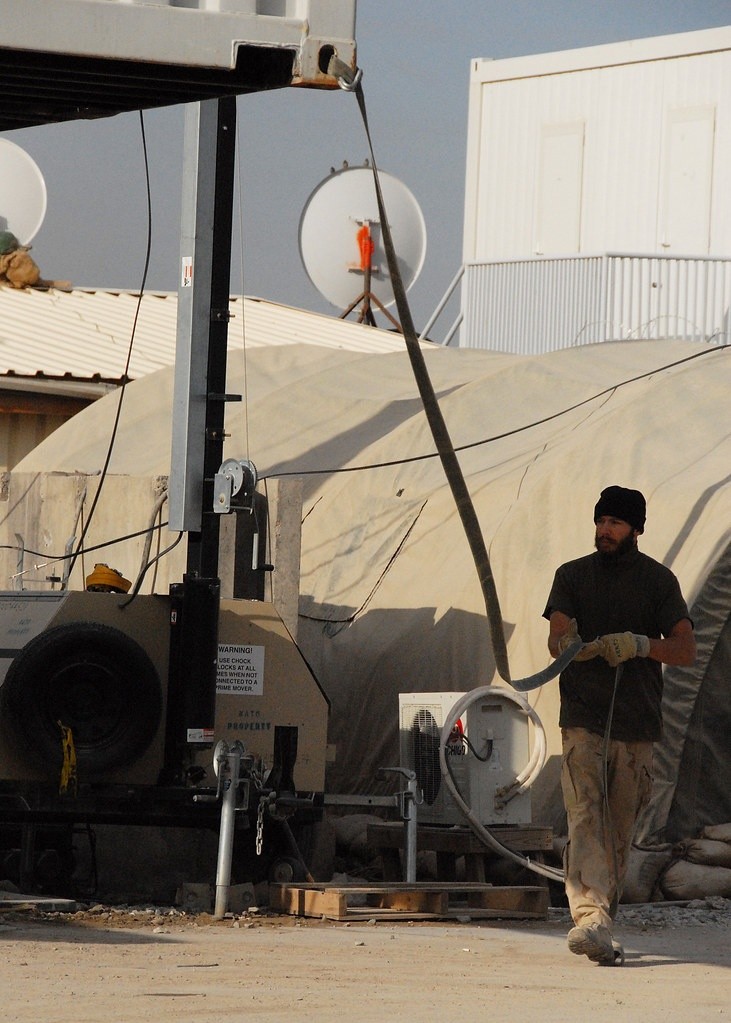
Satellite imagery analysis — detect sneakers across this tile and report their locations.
[567,922,615,965]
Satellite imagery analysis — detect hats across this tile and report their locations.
[593,485,647,535]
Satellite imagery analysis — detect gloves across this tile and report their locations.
[598,631,650,666]
[558,617,606,661]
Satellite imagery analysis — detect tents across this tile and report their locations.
[8,343,731,898]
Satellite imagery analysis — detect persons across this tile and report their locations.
[543,485,695,966]
